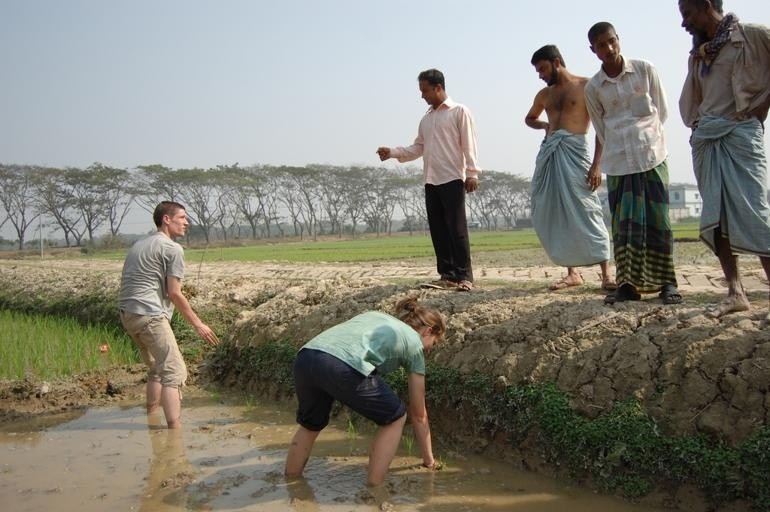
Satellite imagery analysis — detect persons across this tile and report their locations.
[284,294,447,488]
[374,67,482,292]
[520,43,618,293]
[583,22,684,305]
[116,201,221,429]
[677,1,769,321]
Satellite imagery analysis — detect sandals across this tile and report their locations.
[659,285,683,303]
[459,280,473,291]
[434,279,458,287]
[617,283,641,301]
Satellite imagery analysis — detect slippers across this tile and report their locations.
[601,279,616,290]
[550,282,582,290]
